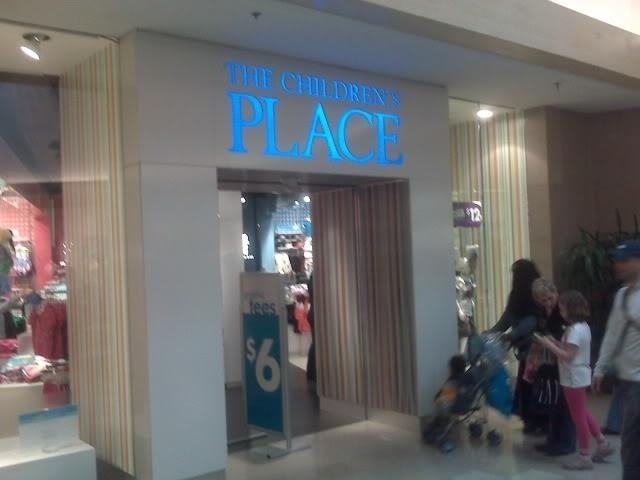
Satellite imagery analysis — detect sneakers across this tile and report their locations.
[561,443,616,471]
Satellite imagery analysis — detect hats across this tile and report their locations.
[606,241,639,258]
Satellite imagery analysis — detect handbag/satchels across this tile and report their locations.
[530,368,562,412]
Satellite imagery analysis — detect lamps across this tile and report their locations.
[477,103,494,119]
[20,32,44,61]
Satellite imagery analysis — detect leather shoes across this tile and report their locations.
[536,443,576,457]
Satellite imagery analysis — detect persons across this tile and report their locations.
[590,239,640,480]
[497,279,577,456]
[600,378,625,437]
[480,256,556,433]
[432,352,471,412]
[0,226,16,296]
[530,291,616,470]
[0,298,28,341]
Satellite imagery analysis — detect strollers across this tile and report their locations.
[418,333,501,453]
